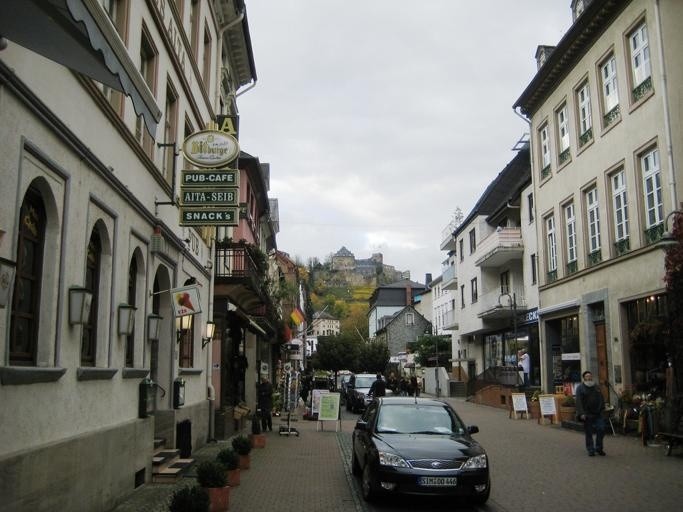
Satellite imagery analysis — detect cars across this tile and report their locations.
[333,370,490,503]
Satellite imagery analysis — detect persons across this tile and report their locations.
[386,373,423,397]
[256,376,273,433]
[631,370,647,419]
[367,371,386,401]
[517,348,531,387]
[576,371,607,457]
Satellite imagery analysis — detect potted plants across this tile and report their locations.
[527,390,544,420]
[559,395,576,422]
[166,408,264,512]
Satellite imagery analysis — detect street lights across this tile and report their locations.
[495,293,519,387]
[424,323,439,367]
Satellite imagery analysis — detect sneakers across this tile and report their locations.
[588,448,606,456]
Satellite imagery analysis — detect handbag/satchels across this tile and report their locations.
[575,411,592,423]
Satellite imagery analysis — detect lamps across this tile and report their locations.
[69,282,92,330]
[116,301,162,343]
[176,314,192,344]
[0,256,17,309]
[202,320,215,349]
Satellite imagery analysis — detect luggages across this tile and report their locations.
[252,405,261,435]
